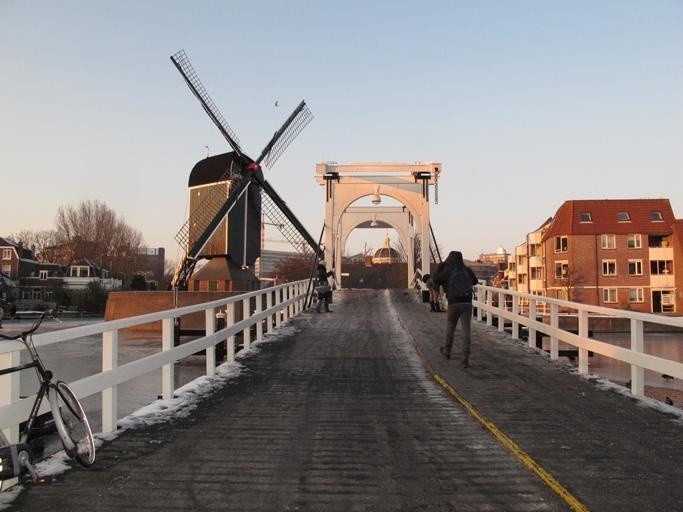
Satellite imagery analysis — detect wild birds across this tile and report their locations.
[626,378,632,388]
[274,101,279,106]
[661,373,674,382]
[664,395,673,405]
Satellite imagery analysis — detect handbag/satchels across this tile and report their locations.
[315,284,331,294]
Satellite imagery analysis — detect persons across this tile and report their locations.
[314,260,335,313]
[432,250,479,368]
[0,305,4,328]
[9,303,17,321]
[426,276,443,312]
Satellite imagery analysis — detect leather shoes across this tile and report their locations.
[440,346,450,360]
[461,360,469,368]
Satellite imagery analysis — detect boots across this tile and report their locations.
[325,304,333,312]
[435,302,443,312]
[430,301,436,312]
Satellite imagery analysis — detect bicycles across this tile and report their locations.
[0,306,96,495]
